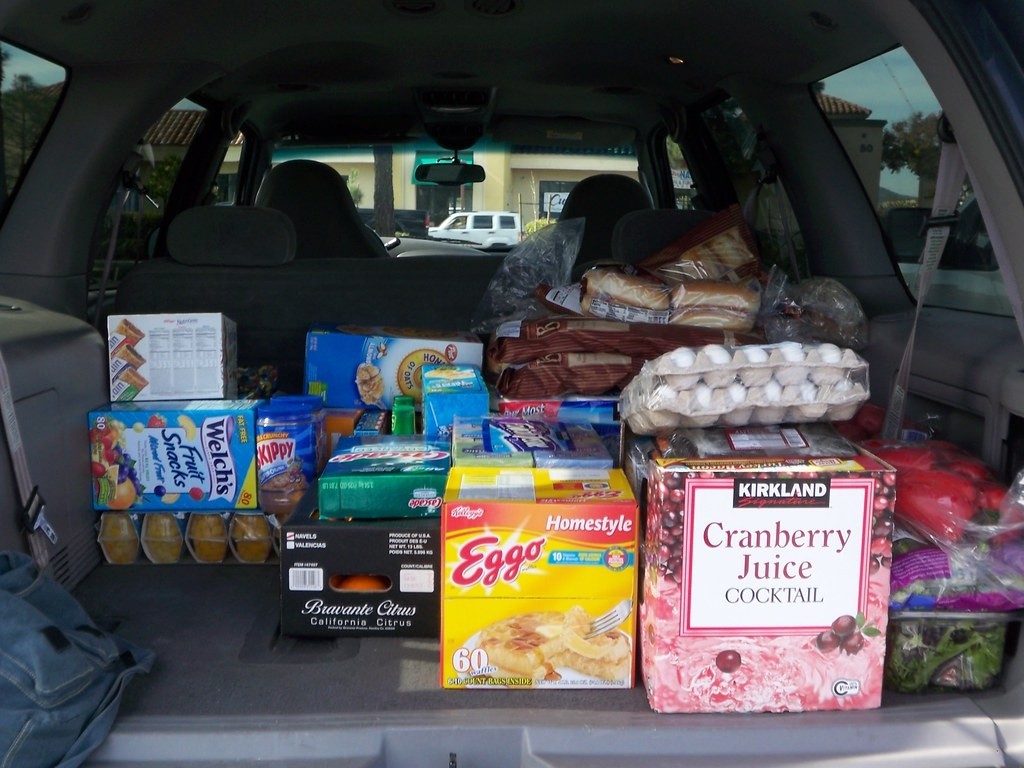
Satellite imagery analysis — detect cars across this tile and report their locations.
[885,193,1000,272]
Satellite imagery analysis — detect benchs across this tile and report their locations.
[117,207,716,367]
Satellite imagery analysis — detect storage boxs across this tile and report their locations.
[421,363,491,438]
[438,465,637,690]
[108,313,238,402]
[325,395,416,458]
[625,433,896,714]
[450,417,615,471]
[87,398,264,510]
[277,480,440,639]
[318,434,450,518]
[302,320,483,412]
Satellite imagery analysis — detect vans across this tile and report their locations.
[427,211,521,250]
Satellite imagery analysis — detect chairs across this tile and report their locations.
[547,174,656,265]
[257,160,391,258]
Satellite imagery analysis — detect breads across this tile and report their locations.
[579,229,760,335]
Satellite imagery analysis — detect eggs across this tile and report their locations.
[649,343,852,407]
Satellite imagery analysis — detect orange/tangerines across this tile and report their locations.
[340,576,385,591]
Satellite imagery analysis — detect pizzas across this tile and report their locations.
[476,614,630,685]
